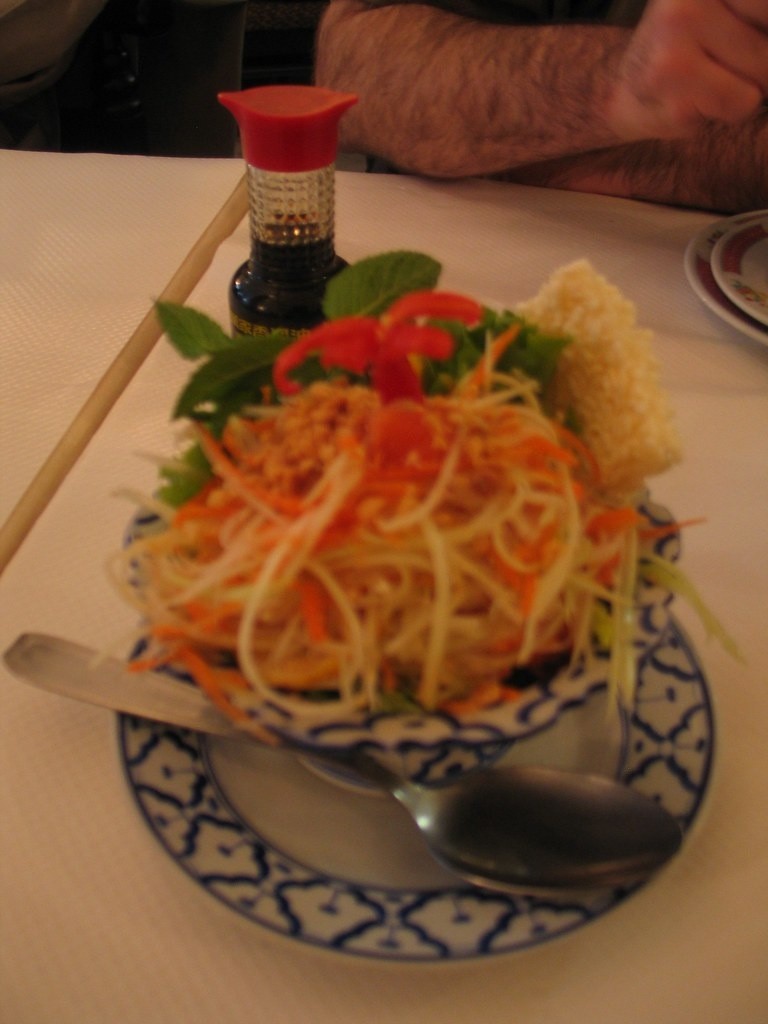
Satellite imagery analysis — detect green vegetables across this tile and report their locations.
[150,249,579,497]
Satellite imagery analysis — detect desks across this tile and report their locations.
[0,147,768,1024]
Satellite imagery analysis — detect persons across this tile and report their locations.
[316,0,768,212]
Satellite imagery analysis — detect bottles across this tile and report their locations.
[217,85,359,344]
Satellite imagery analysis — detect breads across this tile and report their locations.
[507,258,684,486]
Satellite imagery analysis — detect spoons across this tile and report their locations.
[3,633,683,898]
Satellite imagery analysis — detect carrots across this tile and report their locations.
[129,321,706,746]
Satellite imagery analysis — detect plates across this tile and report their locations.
[682,209,768,351]
[117,504,716,959]
